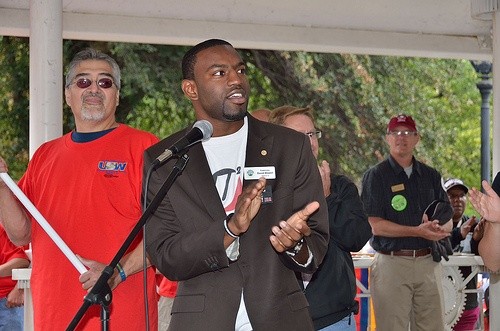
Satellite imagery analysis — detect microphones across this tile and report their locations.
[152,119,214,166]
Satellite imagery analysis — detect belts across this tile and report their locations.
[377,249,431,256]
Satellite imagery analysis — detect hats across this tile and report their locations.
[387,114,417,132]
[442,175,468,193]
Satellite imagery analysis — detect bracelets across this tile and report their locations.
[224,212,244,238]
[116,262,127,282]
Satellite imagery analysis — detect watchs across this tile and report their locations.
[287,235,305,258]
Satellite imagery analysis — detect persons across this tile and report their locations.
[0,48,162,331]
[143,39,331,331]
[359,114,455,331]
[444,179,487,331]
[0,223,31,331]
[153,268,179,331]
[252,109,274,123]
[468,172,500,272]
[266,105,374,331]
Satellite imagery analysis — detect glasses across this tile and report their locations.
[66,77,119,89]
[306,130,323,139]
[388,129,416,135]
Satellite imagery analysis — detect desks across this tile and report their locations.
[12,251,486,331]
